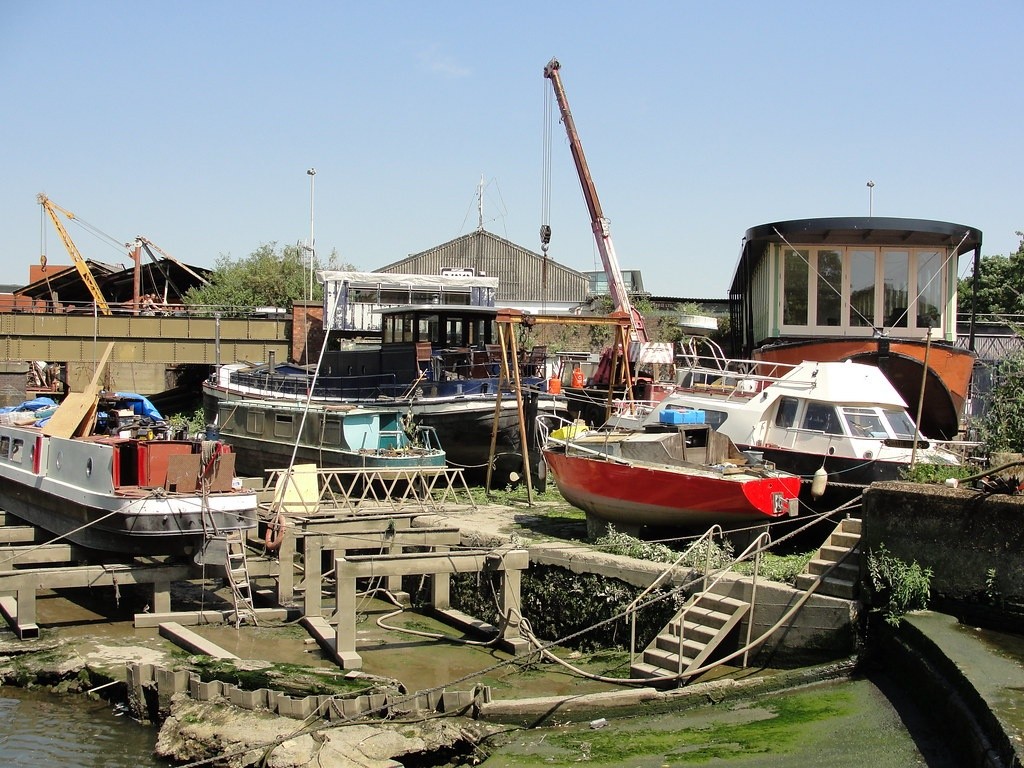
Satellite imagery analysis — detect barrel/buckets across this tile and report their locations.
[743,450,764,465]
[549,375,560,394]
[571,368,583,387]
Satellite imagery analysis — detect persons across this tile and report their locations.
[143,294,158,311]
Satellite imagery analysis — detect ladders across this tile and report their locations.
[224,529,258,631]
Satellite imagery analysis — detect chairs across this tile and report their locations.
[521,345,547,379]
[416,342,434,382]
[482,344,502,379]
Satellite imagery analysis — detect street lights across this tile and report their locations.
[306,167,316,301]
[866,181,875,217]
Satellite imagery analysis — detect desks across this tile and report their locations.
[97,417,115,436]
[449,347,486,379]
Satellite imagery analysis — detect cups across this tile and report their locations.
[946,479,959,488]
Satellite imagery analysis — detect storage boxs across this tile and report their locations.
[660,409,706,424]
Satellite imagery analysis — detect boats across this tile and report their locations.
[0,397,260,556]
[204,398,446,498]
[201,168,995,532]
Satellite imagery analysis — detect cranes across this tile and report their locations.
[37,189,131,315]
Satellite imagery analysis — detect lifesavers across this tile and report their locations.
[266,516,286,548]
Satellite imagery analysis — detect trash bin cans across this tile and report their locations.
[206,424,220,441]
[743,450,764,465]
